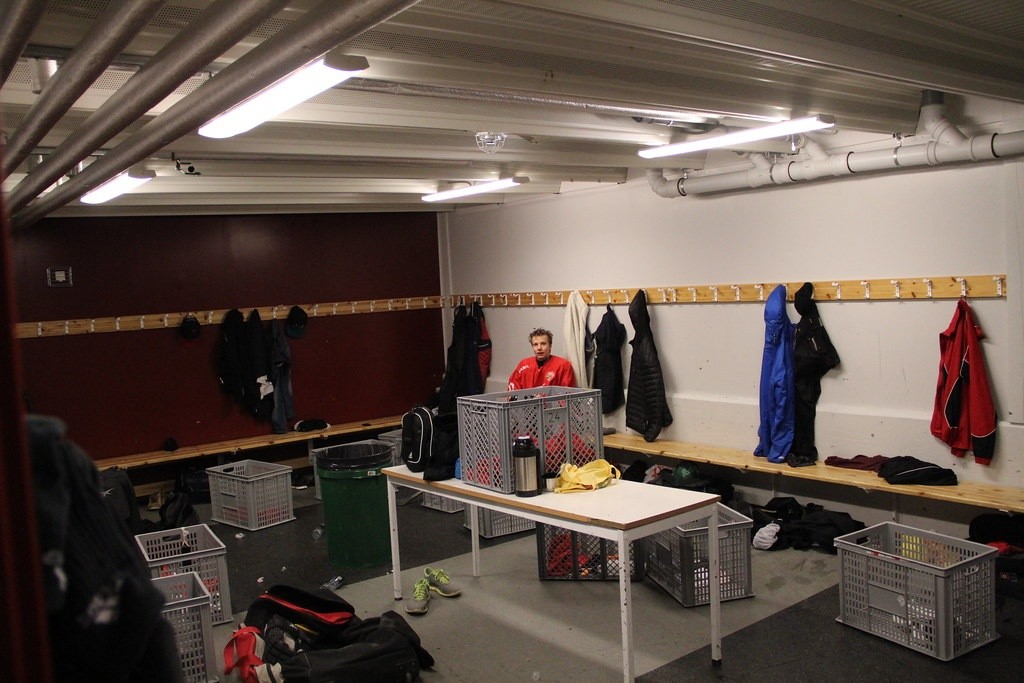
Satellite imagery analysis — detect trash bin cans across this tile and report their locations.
[315,440,395,570]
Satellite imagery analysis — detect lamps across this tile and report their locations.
[474,132,508,155]
[46,266,73,288]
[81,167,157,204]
[638,114,836,159]
[422,176,530,202]
[198,55,371,138]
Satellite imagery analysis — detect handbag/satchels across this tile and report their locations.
[222,622,285,683]
[266,608,435,683]
[401,403,435,472]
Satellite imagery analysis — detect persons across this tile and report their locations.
[508,327,577,439]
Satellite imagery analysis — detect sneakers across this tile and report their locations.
[424,566,462,596]
[405,579,432,613]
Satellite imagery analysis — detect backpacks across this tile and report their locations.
[237,582,363,647]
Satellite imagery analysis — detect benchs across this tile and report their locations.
[591,432,1024,514]
[94,415,404,471]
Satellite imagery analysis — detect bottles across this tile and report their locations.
[319,576,345,592]
[312,520,326,540]
[512,435,542,497]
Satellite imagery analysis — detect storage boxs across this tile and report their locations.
[463,503,537,538]
[458,386,605,495]
[310,430,423,505]
[834,520,1001,661]
[135,523,233,627]
[535,521,645,582]
[644,502,757,609]
[421,491,465,513]
[206,459,297,532]
[150,571,220,683]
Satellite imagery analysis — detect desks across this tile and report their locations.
[381,463,722,683]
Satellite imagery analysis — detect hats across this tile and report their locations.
[286,306,307,337]
